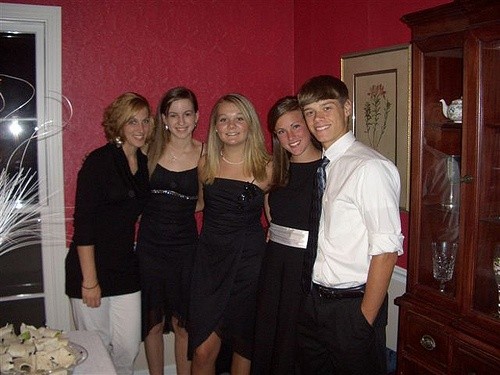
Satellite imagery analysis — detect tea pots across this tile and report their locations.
[436,95,470,130]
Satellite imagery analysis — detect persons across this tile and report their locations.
[186,93,274,375]
[65,91,152,375]
[294,75,404,375]
[249,94,322,375]
[81,87,207,375]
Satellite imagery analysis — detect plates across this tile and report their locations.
[0,337,87,374]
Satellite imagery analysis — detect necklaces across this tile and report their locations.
[220,150,244,164]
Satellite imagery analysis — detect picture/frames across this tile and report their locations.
[339,43,412,213]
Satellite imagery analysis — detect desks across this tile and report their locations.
[59,331,116,375]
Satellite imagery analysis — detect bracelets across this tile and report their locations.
[81,282,100,290]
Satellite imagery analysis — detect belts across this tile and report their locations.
[310,283,366,301]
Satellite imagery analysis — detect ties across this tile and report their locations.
[316,156,330,199]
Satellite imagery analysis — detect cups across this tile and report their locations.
[426,241,462,287]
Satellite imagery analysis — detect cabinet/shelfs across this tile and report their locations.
[394,0,500,375]
